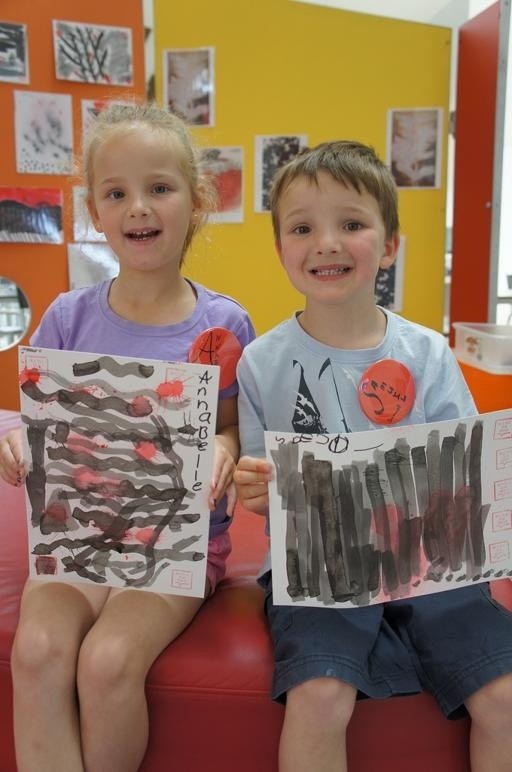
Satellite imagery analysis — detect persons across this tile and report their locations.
[232,141,512,772]
[0,100,255,770]
